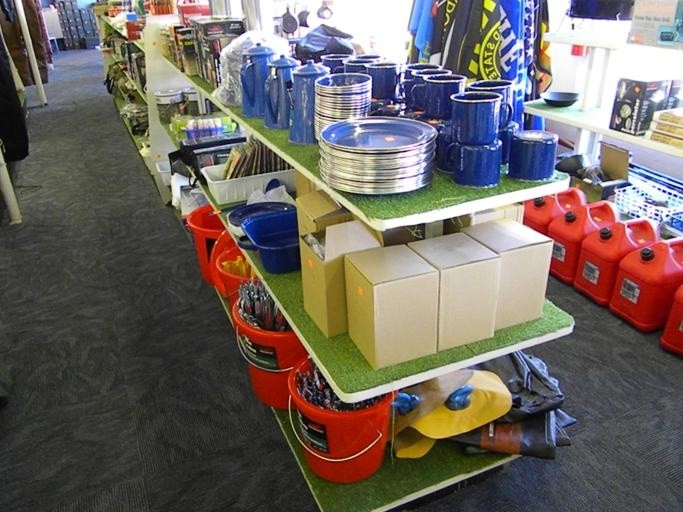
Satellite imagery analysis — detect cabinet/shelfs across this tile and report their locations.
[522,76,683,240]
[97,14,577,512]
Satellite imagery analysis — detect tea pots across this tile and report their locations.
[241,43,331,145]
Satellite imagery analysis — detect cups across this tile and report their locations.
[322,54,559,190]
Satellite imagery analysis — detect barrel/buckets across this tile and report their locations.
[286,354,397,485]
[523,188,683,357]
[153,89,185,124]
[181,87,199,116]
[180,203,225,286]
[207,230,299,411]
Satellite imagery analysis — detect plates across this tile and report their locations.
[228,201,298,238]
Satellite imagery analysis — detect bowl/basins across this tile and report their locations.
[539,91,580,107]
[555,155,584,176]
[236,211,301,277]
